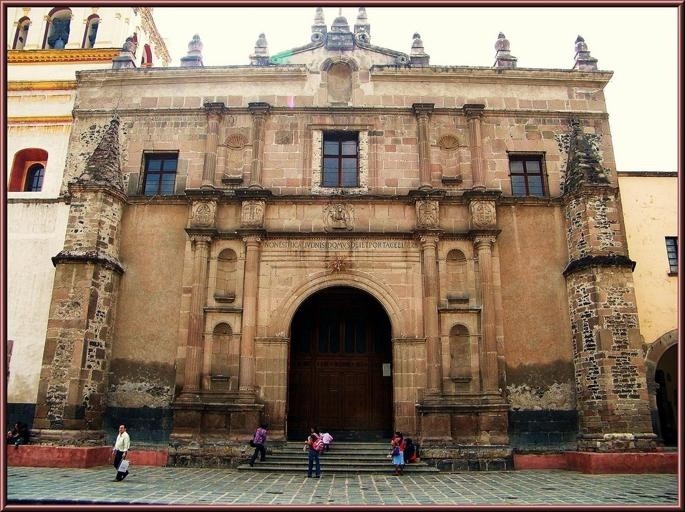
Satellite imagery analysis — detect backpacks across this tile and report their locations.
[310,433,325,453]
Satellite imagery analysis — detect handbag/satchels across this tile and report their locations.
[118,457,130,472]
[250,440,256,447]
[393,447,399,456]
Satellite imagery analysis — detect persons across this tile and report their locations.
[319,431,333,454]
[403,438,415,464]
[390,432,407,476]
[111,424,130,482]
[304,427,320,478]
[248,423,268,467]
[7,421,29,445]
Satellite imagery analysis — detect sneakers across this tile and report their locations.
[248,459,267,467]
[308,475,320,478]
[392,473,404,476]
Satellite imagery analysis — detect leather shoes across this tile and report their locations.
[110,471,129,482]
